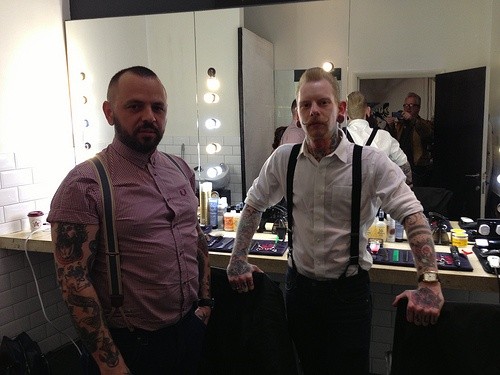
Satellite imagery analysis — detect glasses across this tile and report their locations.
[403,103,420,108]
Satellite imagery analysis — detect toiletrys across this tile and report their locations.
[199,181,240,231]
[368,211,411,243]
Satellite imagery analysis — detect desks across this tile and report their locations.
[0,224,500,375]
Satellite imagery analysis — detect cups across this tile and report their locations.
[27,211,44,234]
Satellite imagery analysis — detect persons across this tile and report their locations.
[270,126,288,154]
[47,65,215,375]
[386,93,434,186]
[227,67,443,375]
[279,98,305,145]
[342,91,414,189]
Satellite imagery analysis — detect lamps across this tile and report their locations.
[205,143,221,154]
[294,62,341,82]
[207,166,222,178]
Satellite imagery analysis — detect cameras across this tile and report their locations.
[392,110,404,120]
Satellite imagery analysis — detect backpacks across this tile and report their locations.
[0,331,50,375]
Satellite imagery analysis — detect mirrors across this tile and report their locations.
[65,0,500,244]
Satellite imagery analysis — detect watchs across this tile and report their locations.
[197,298,214,310]
[418,271,441,284]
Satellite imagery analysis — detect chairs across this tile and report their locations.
[412,187,453,221]
[385,296,500,375]
[208,266,300,375]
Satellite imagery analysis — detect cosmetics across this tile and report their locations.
[368,213,468,247]
[199,184,240,232]
